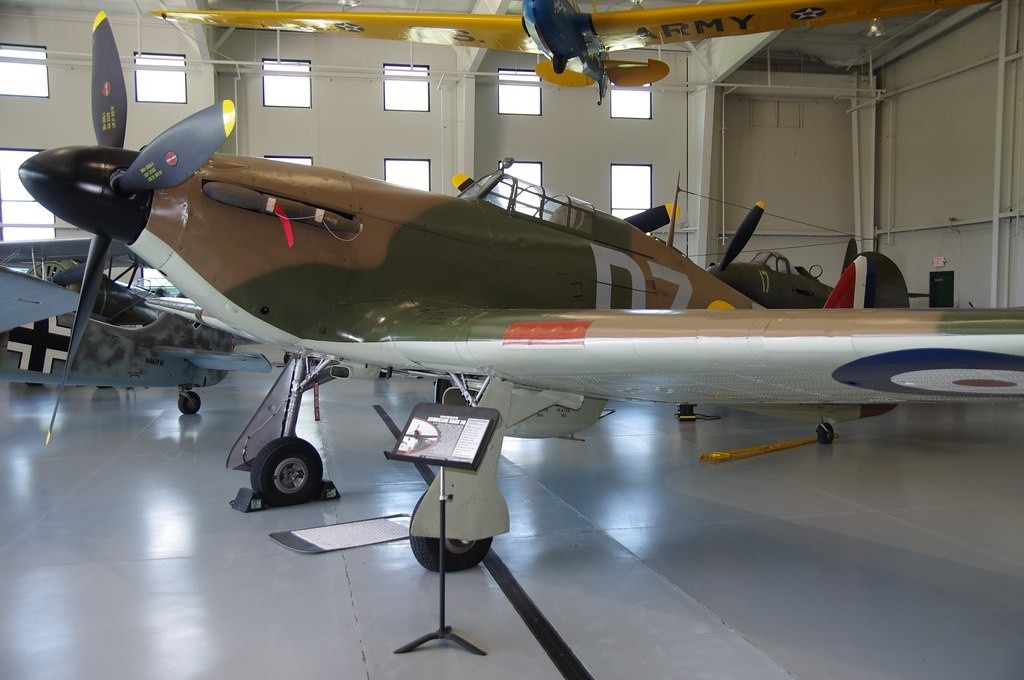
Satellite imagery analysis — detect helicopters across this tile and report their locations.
[17,0,1024,573]
[0,237,270,415]
